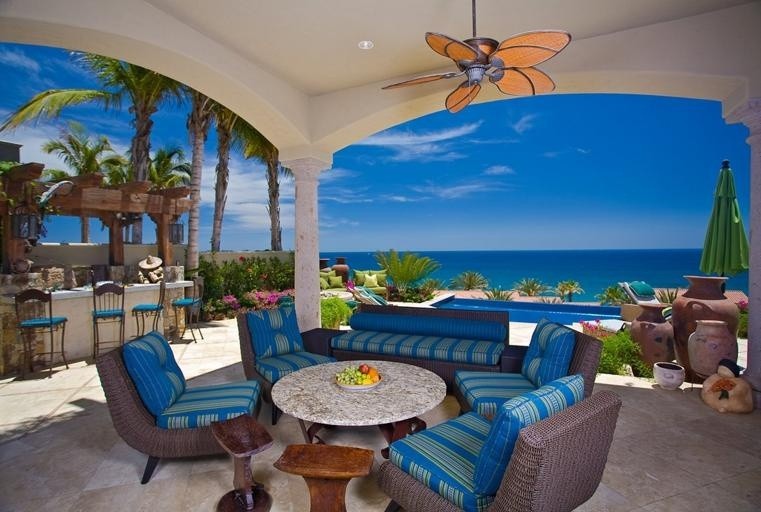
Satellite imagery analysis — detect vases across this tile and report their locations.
[652,362,686,391]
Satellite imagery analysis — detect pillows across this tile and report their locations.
[353,268,387,287]
[349,311,507,342]
[319,269,345,289]
[246,304,306,360]
[629,281,655,298]
[521,319,577,390]
[472,373,584,496]
[122,330,186,417]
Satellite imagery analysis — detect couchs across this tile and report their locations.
[330,302,509,395]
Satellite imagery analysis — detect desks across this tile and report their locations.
[321,291,353,301]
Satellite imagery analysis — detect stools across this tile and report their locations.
[273,444,375,512]
[210,415,274,512]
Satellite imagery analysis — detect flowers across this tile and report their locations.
[224,255,295,314]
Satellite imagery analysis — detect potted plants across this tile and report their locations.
[1,119,192,199]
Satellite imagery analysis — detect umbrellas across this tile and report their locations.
[700,157,752,292]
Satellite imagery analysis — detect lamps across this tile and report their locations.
[169,224,184,244]
[11,213,39,240]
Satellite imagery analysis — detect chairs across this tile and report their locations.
[455,322,604,421]
[133,282,167,338]
[94,335,263,484]
[348,286,437,309]
[91,283,126,357]
[236,308,338,424]
[376,389,622,512]
[617,281,672,324]
[169,277,205,343]
[323,287,347,291]
[14,288,68,379]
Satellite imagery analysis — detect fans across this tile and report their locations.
[381,0,573,113]
